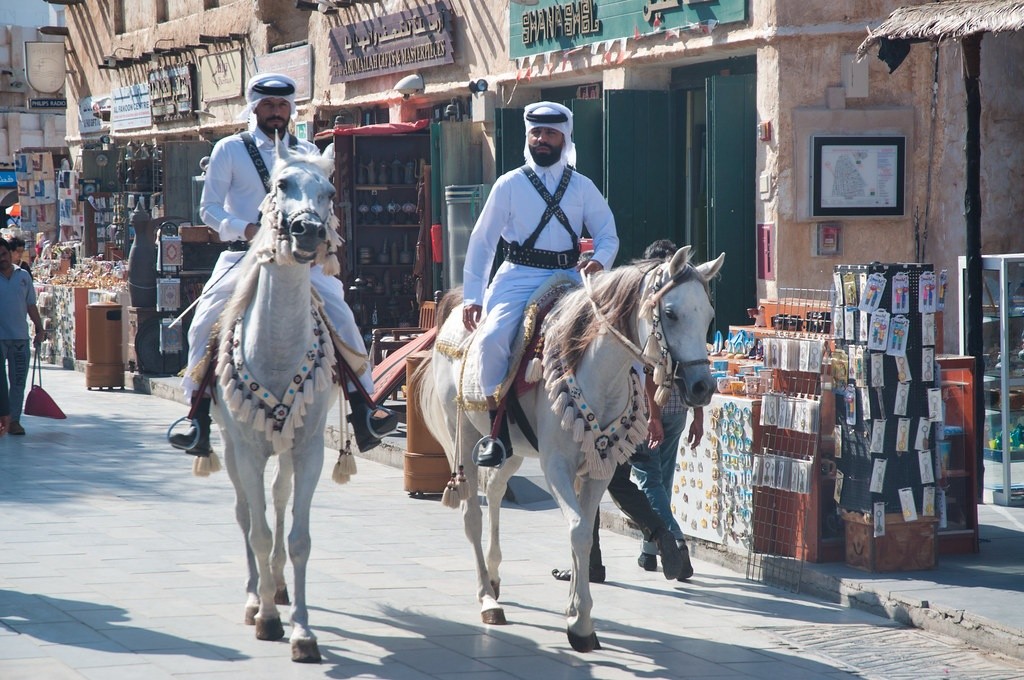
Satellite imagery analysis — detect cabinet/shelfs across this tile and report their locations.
[353,134,431,321]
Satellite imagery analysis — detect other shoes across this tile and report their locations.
[7,421,26,435]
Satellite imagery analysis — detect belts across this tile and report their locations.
[226,241,250,251]
[505,243,579,271]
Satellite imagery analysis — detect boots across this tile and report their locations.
[169,397,211,458]
[476,410,513,467]
[345,393,398,454]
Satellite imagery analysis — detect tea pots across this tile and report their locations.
[357,153,426,186]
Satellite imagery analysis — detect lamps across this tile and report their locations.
[468,80,488,94]
[104,43,133,67]
[144,37,174,62]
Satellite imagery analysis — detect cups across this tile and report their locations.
[711,361,773,398]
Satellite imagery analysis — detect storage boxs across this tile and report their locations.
[844,513,935,570]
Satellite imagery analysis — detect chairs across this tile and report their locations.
[369,301,435,401]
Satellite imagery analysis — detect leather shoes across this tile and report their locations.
[673,540,694,579]
[657,531,680,581]
[637,553,658,571]
[552,567,605,585]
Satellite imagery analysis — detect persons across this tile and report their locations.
[463,102,620,465]
[171,73,398,457]
[10,236,34,281]
[553,360,684,582]
[0,237,46,436]
[638,238,704,580]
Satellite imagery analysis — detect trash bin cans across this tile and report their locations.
[86,302,125,390]
[403,352,451,497]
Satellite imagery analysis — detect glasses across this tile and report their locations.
[15,249,25,254]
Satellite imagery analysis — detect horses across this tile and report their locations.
[409,246,730,652]
[185,125,339,665]
